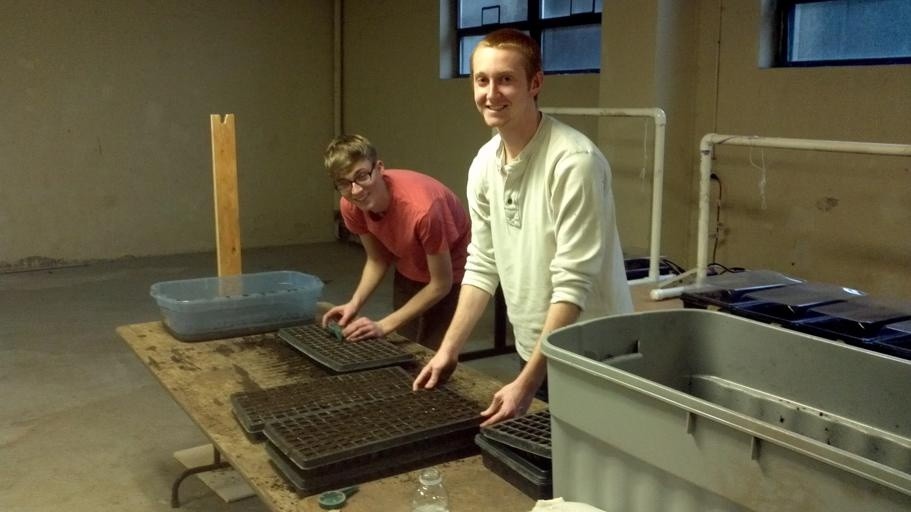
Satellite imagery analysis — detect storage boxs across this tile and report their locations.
[144,270,324,342]
[540,308,909,509]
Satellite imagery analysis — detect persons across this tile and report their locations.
[322,130,470,379]
[411,30,637,430]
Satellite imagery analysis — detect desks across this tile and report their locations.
[115,299,559,510]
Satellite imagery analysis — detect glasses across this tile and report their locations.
[334,163,375,191]
